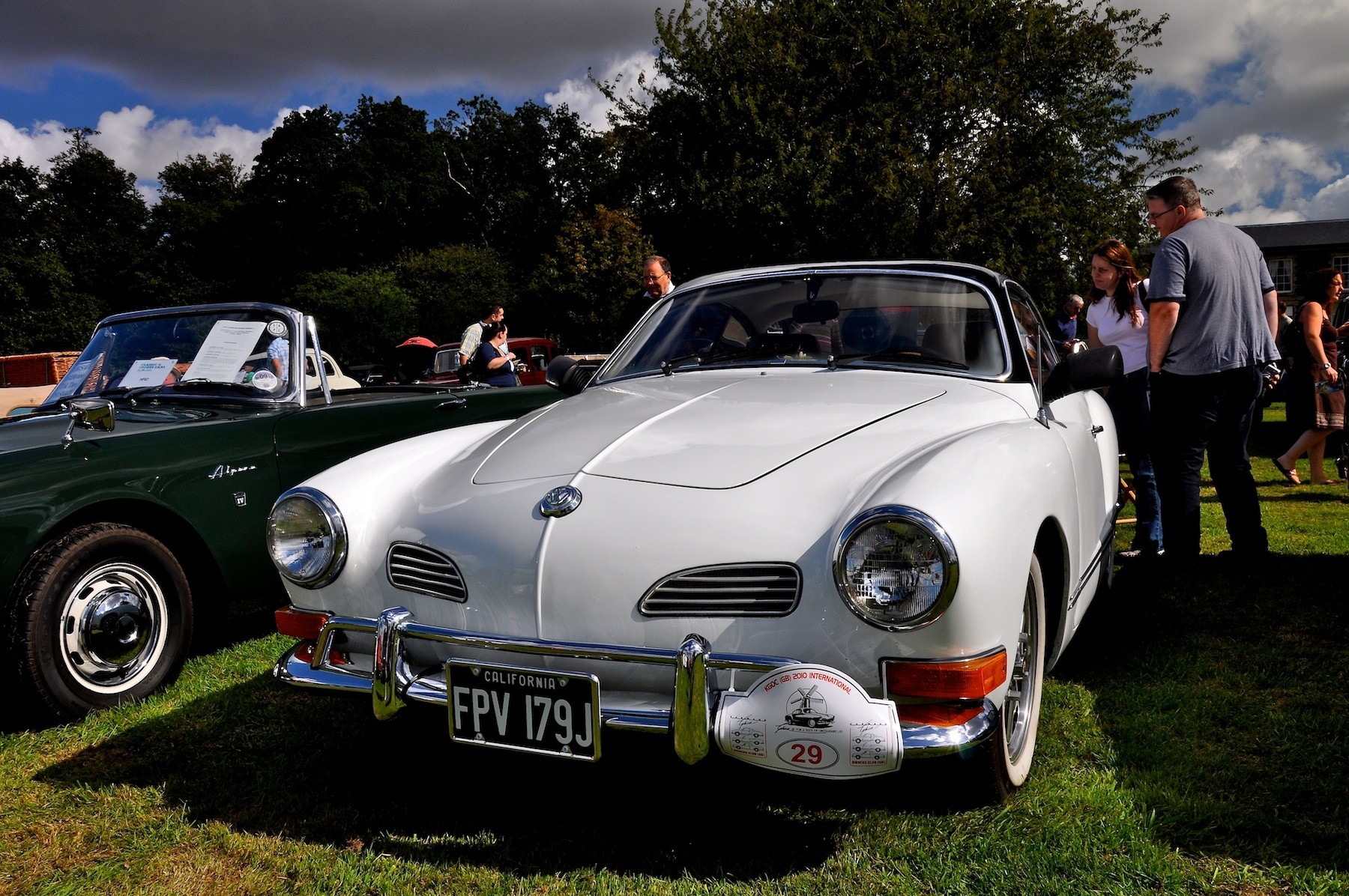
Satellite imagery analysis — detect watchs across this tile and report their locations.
[1318,363,1330,370]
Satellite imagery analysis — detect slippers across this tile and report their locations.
[1273,457,1300,486]
[1312,476,1339,485]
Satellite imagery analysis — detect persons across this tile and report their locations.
[880,279,919,312]
[788,315,847,358]
[1086,238,1170,558]
[263,319,309,382]
[1278,268,1349,486]
[1054,295,1085,356]
[458,305,527,387]
[1142,177,1283,561]
[616,255,699,377]
[244,362,258,384]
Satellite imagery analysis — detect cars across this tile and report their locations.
[411,335,555,392]
[257,255,1127,810]
[0,300,574,732]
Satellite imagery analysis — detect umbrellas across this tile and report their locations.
[396,336,438,348]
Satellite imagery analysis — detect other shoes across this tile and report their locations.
[1218,550,1260,568]
[1117,544,1159,562]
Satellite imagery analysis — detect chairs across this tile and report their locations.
[746,333,821,357]
[923,322,1004,375]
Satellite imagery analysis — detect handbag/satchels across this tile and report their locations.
[1283,300,1325,351]
[457,351,477,384]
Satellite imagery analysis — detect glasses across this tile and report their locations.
[1149,203,1189,221]
[1071,302,1081,311]
[641,273,664,281]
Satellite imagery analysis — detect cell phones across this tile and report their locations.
[1262,365,1281,379]
